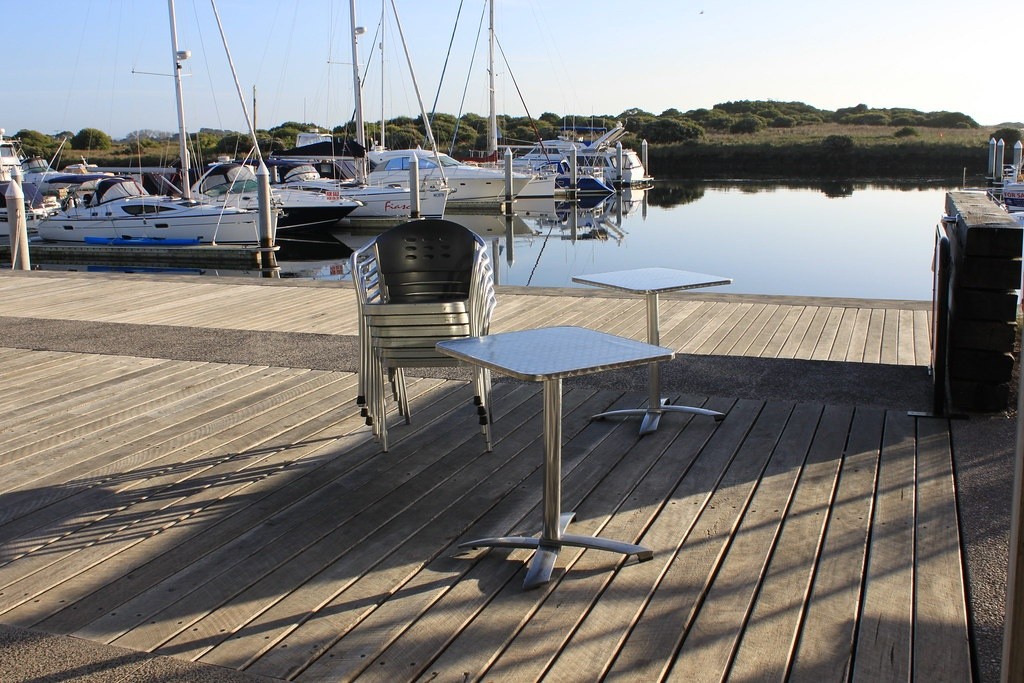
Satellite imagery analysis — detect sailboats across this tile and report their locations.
[0,0,655,257]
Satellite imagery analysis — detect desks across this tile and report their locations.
[436,325,677,587]
[573,268,733,435]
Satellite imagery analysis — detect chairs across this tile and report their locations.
[350,219,497,454]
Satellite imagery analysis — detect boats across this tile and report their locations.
[987,162,1024,227]
